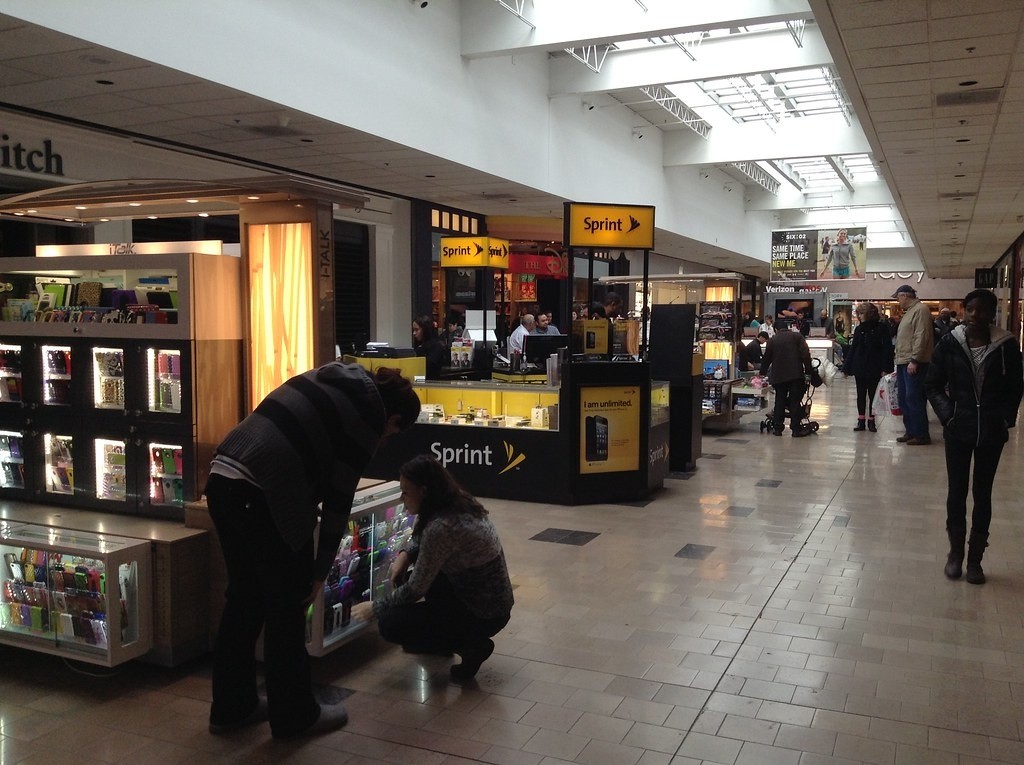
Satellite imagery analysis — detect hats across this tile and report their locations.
[891,285,915,298]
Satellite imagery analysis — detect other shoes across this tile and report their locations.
[449,637,495,679]
[208,696,270,735]
[791,430,799,437]
[897,433,912,442]
[907,435,931,445]
[772,430,782,436]
[272,703,348,740]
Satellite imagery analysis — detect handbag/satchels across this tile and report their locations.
[870,371,902,416]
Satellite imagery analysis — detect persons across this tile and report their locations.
[822,237,830,265]
[891,285,934,445]
[603,292,623,316]
[790,311,810,336]
[205,361,422,738]
[412,315,464,379]
[934,307,958,337]
[814,309,834,335]
[745,311,760,327]
[350,453,515,681]
[836,329,848,345]
[841,303,894,432]
[592,302,613,361]
[836,311,845,334]
[451,353,471,367]
[819,229,861,279]
[859,235,864,250]
[738,331,769,371]
[924,289,1024,583]
[759,315,776,338]
[759,320,812,437]
[510,311,561,353]
[572,305,588,320]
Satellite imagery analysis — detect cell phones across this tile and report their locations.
[586,415,608,462]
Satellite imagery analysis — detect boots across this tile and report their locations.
[944,527,966,579]
[967,528,990,584]
[854,418,866,431]
[868,419,877,432]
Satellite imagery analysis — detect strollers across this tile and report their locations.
[760,357,821,434]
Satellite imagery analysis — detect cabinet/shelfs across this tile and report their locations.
[0,252,773,666]
